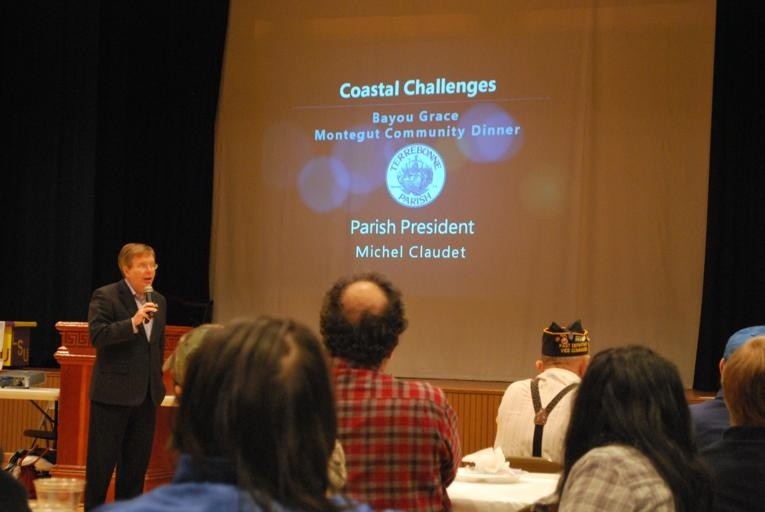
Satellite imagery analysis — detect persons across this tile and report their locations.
[96,311,370,512]
[83,242,168,512]
[698,336,764,510]
[686,326,764,443]
[554,344,715,511]
[495,320,591,467]
[159,322,347,493]
[320,273,462,512]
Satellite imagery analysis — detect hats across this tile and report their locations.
[724,325,765,361]
[541,318,589,358]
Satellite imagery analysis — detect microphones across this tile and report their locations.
[144,285,154,317]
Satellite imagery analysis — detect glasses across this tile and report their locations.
[140,263,159,270]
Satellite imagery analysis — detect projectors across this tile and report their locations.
[0,370,45,387]
